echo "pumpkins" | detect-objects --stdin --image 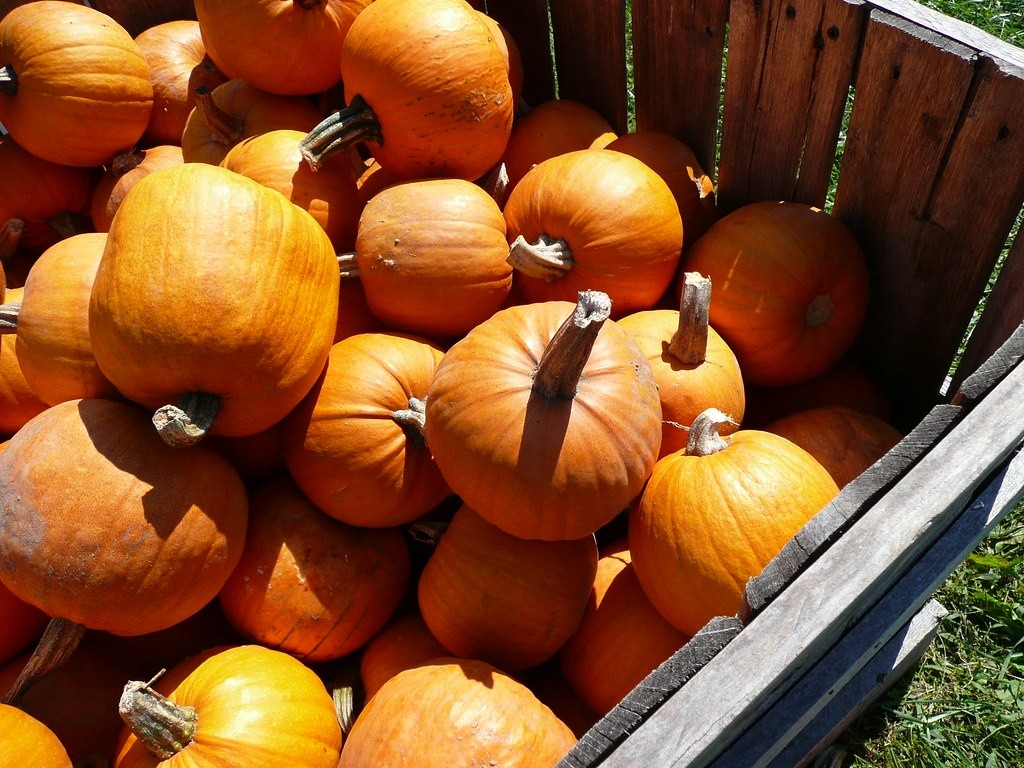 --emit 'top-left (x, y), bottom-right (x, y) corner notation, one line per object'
(0, 0), (915, 768)
